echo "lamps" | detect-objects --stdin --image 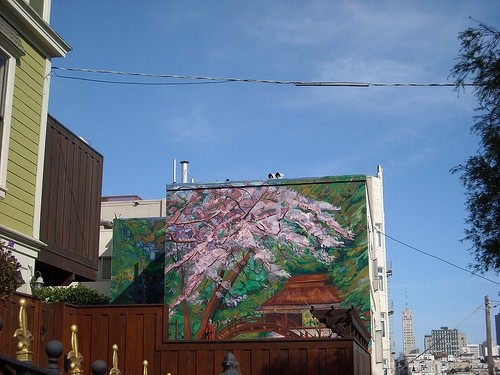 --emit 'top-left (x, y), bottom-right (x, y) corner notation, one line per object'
(34, 270), (45, 291)
